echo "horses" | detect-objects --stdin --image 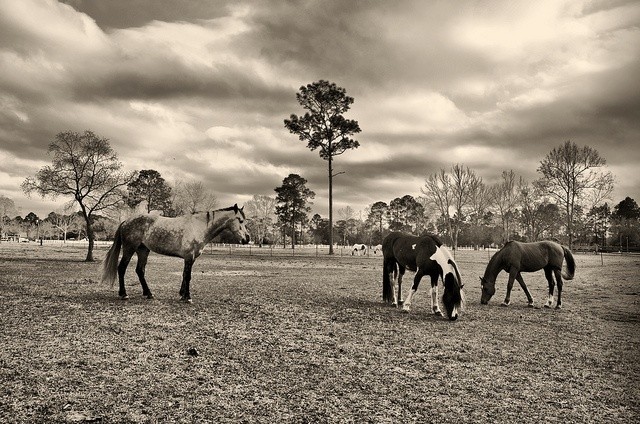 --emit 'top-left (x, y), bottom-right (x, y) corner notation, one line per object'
(373, 244), (382, 255)
(478, 241), (576, 310)
(95, 203), (251, 305)
(382, 230), (467, 321)
(351, 244), (367, 256)
